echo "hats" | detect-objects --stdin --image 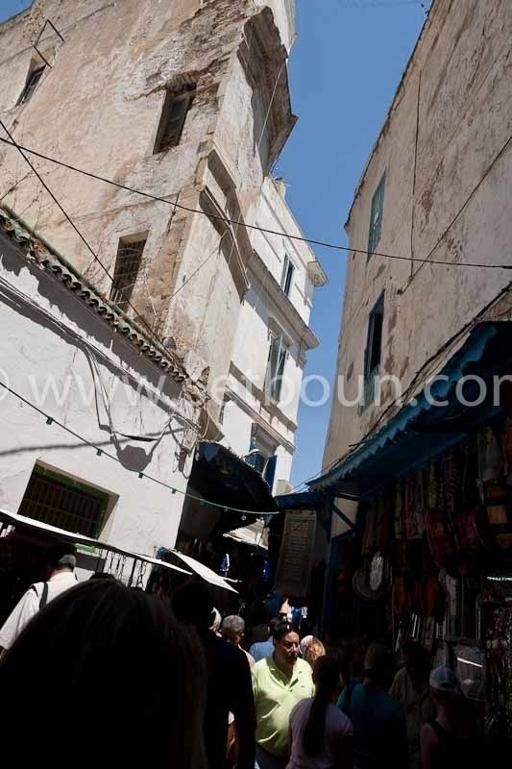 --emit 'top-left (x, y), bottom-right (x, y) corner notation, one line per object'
(427, 666), (459, 692)
